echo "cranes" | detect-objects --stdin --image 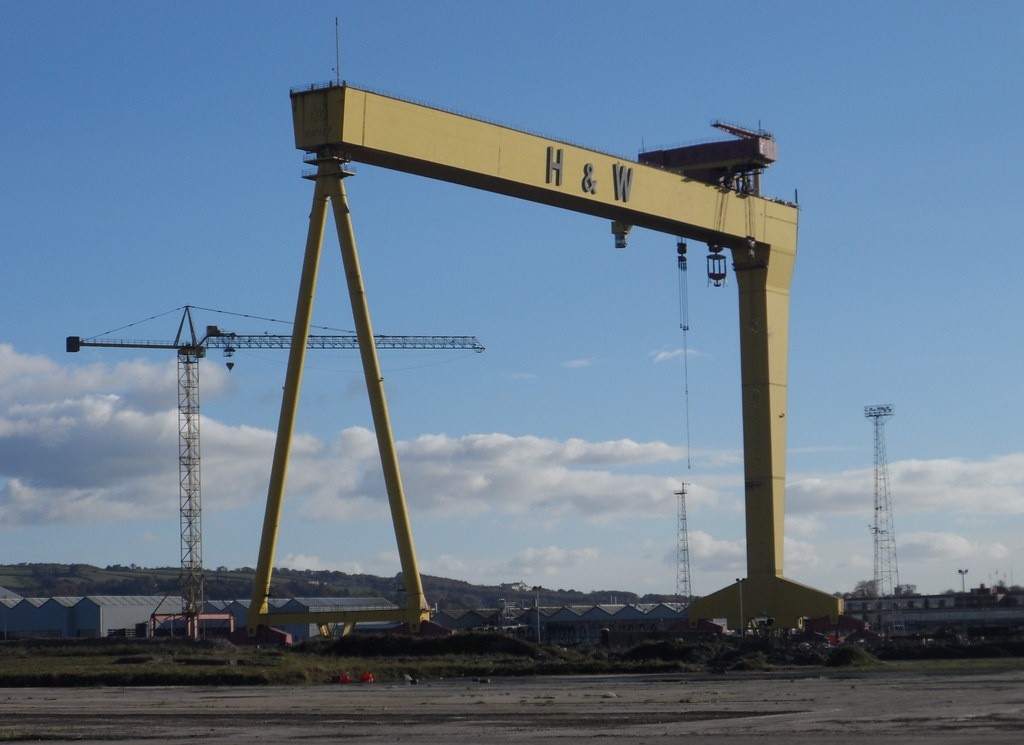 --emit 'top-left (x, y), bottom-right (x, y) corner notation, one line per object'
(66, 304), (487, 617)
